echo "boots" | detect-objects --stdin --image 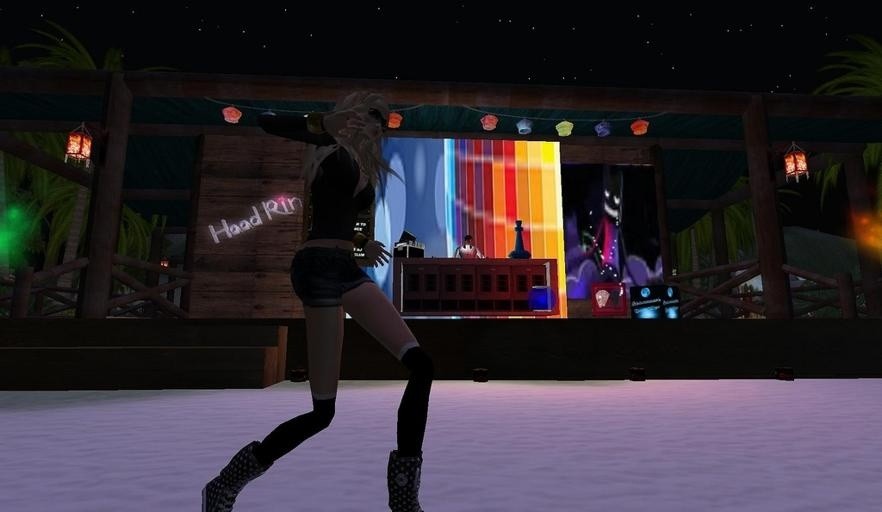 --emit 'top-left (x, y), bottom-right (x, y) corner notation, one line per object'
(201, 441), (274, 512)
(387, 450), (424, 512)
